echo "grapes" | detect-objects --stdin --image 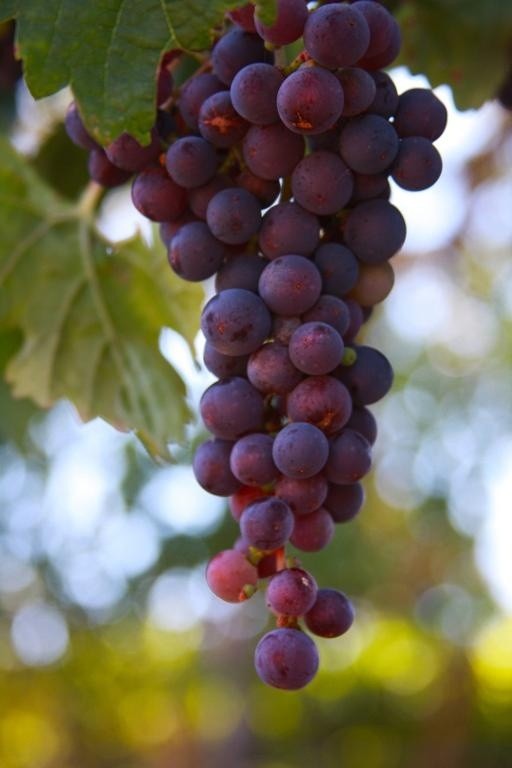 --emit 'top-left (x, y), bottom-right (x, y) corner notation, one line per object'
(64, 0), (447, 691)
